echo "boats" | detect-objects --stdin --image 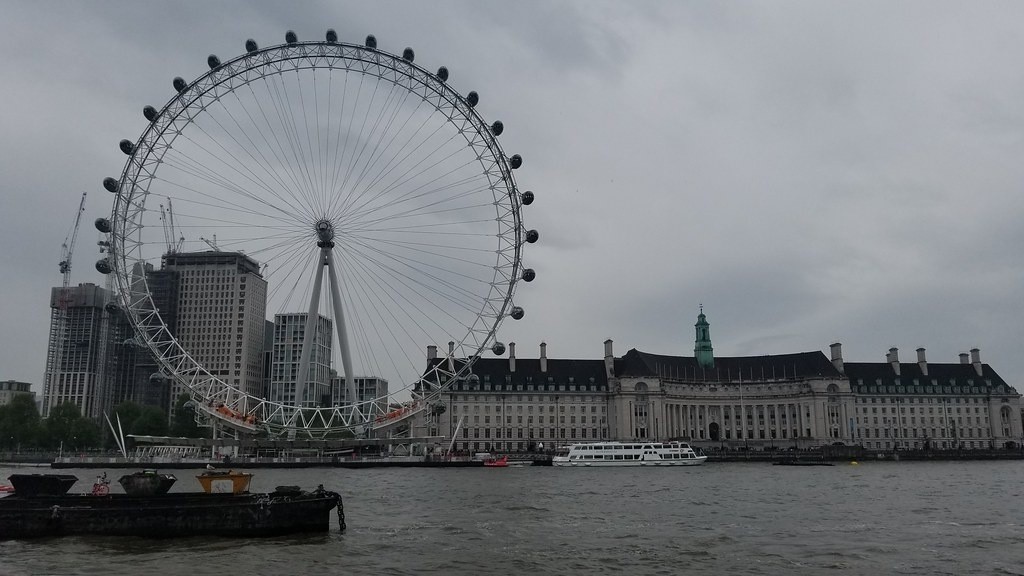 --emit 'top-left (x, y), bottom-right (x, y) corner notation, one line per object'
(484, 456), (534, 467)
(772, 463), (834, 465)
(0, 470), (337, 536)
(552, 441), (708, 466)
